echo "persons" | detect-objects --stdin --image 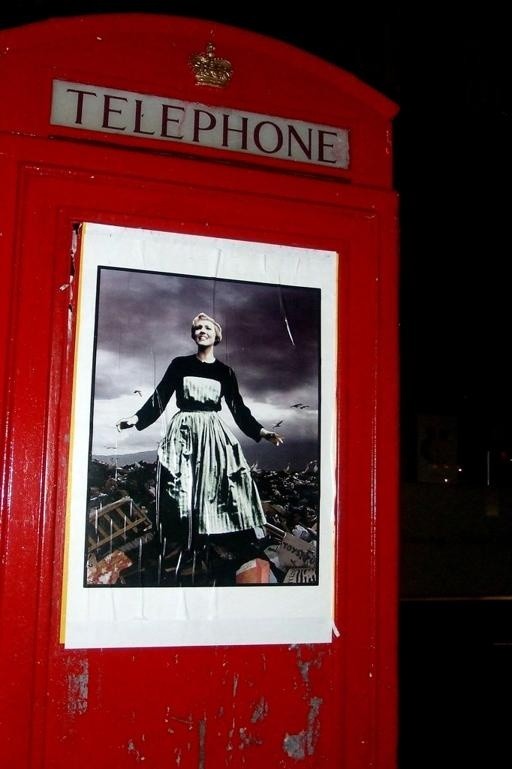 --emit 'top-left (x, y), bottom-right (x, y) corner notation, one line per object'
(114, 311), (286, 567)
